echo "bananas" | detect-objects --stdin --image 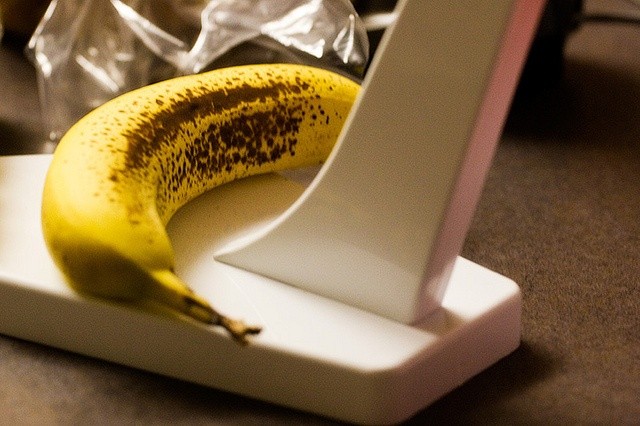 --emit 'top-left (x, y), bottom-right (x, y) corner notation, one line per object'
(39, 62), (366, 349)
